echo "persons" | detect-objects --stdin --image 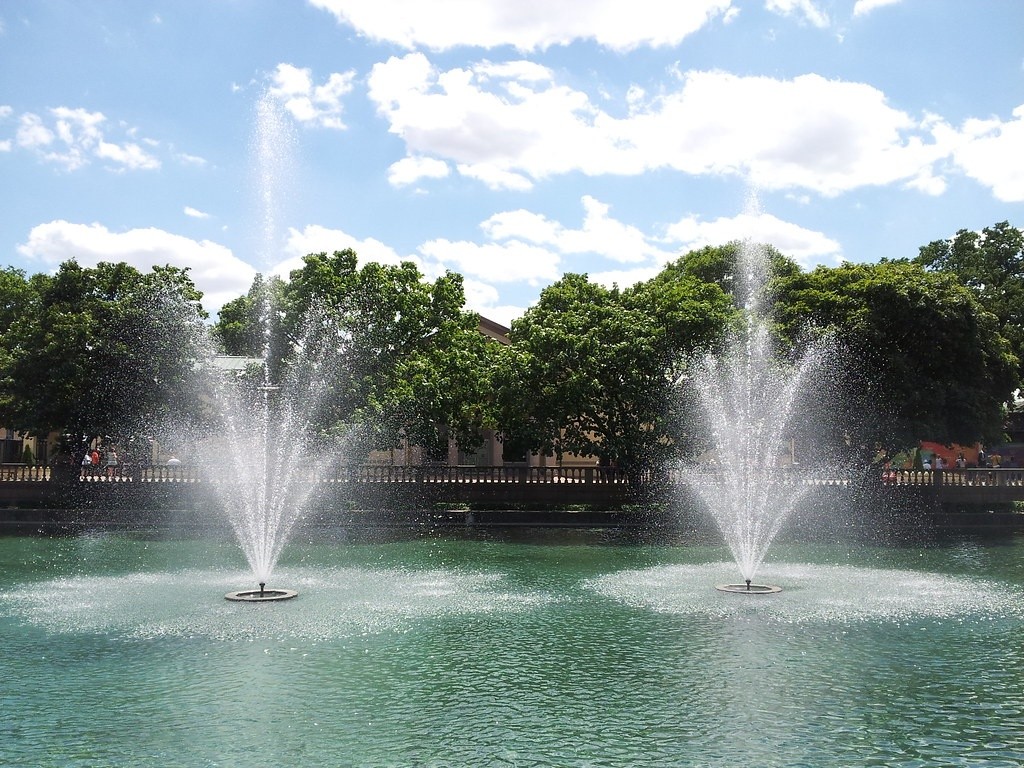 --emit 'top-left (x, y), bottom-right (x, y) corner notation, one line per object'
(81, 447), (117, 480)
(883, 448), (1024, 480)
